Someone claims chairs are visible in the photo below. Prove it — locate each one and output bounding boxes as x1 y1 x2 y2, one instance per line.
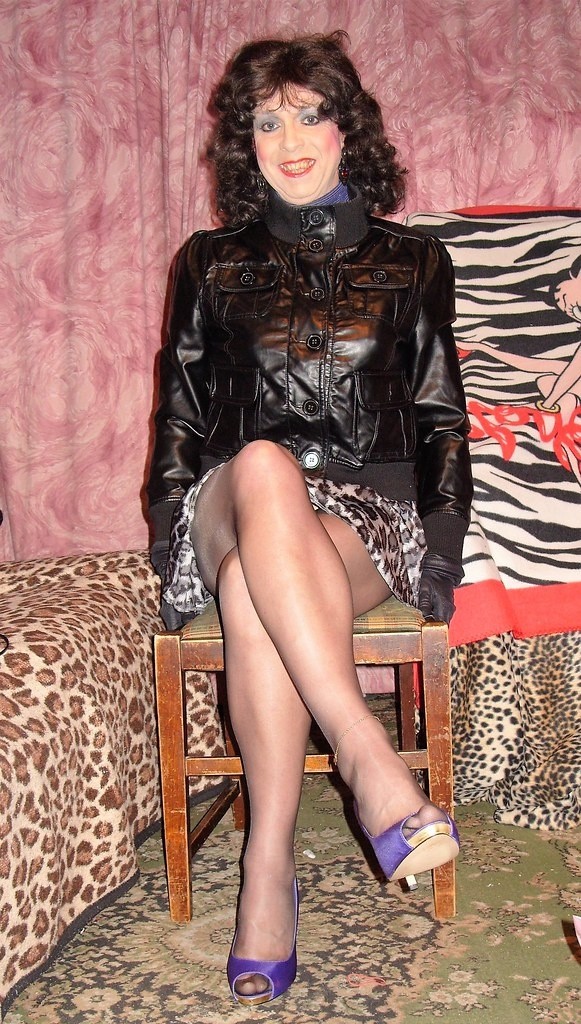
155 593 457 926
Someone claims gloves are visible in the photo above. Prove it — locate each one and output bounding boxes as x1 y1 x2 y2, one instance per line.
420 571 455 627
151 546 193 629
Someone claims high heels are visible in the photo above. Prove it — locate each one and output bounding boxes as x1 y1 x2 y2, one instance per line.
227 876 300 1005
354 797 459 890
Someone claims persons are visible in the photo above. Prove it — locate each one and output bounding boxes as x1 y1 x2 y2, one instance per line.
144 35 474 1008
